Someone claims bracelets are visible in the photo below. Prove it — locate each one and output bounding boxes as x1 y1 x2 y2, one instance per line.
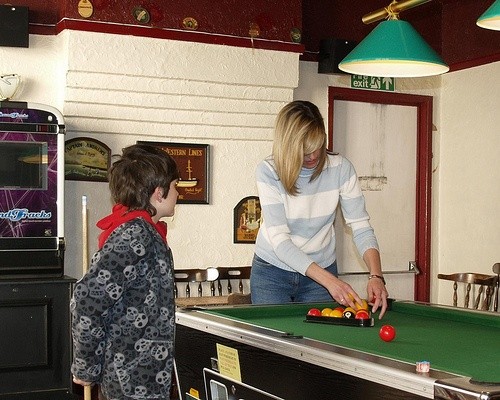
368 275 386 285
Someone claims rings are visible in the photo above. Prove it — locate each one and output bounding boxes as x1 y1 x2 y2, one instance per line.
340 299 345 302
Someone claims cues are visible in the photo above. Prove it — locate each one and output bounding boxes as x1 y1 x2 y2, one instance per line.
82 196 91 400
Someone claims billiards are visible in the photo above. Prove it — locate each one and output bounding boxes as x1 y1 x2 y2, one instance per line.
321 308 333 317
307 308 321 316
329 310 342 317
343 290 370 319
379 324 396 342
334 307 344 312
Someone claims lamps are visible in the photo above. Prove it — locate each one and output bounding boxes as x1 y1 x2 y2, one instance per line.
476 0 500 32
338 15 451 78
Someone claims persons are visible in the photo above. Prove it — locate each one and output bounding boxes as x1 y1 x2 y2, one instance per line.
249 101 389 319
70 144 178 400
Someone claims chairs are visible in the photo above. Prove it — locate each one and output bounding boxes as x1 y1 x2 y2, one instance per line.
438 263 500 312
174 267 251 298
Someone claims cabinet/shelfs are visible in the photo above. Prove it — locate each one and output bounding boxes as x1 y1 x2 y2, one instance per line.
0 274 78 400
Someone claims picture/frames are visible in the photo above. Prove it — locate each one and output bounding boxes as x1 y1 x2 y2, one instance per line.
136 141 210 204
65 137 111 182
233 196 262 244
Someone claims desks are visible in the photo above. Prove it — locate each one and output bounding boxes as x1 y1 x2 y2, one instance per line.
171 298 500 400
